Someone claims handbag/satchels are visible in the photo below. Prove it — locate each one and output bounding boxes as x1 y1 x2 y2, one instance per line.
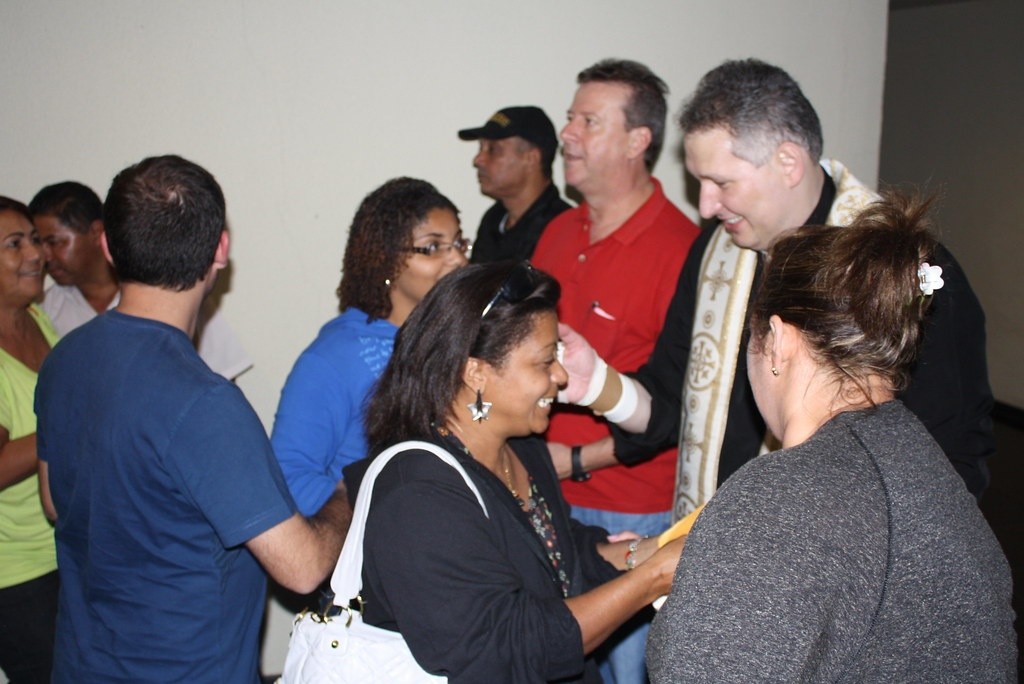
273 443 488 684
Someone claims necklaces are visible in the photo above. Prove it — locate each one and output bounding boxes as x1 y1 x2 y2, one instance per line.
502 458 523 507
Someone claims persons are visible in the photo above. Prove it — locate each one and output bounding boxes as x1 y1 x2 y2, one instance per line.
341 260 685 684
270 176 468 519
27 181 123 337
528 60 705 683
458 105 574 265
554 60 993 502
32 154 353 684
644 186 1018 684
1 193 62 684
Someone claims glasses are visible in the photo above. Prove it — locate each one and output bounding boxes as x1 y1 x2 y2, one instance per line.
400 238 470 258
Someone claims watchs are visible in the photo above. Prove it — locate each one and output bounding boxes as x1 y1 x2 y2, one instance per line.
570 446 592 482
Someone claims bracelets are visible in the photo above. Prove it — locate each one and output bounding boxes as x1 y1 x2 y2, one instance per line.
625 537 644 571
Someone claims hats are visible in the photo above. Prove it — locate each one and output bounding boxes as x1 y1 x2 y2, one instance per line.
459 106 558 154
